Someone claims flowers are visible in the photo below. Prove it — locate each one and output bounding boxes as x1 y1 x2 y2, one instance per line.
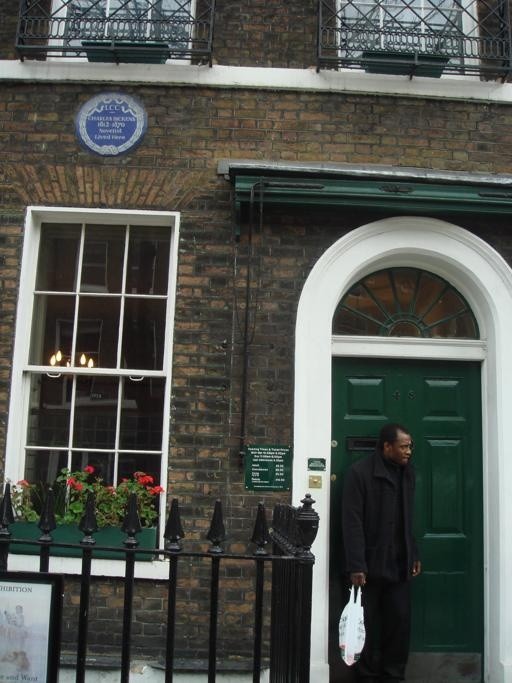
5 466 164 528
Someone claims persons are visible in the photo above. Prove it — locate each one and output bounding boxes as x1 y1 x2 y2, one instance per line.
329 423 421 683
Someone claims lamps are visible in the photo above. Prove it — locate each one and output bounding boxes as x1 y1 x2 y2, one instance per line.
47 350 94 377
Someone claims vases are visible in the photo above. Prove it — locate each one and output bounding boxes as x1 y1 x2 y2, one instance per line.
9 521 157 560
